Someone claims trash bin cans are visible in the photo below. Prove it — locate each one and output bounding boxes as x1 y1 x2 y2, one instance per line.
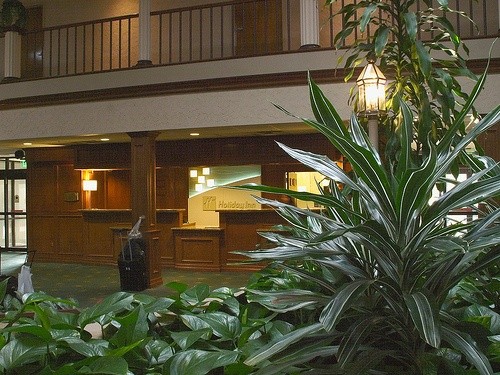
119 239 146 290
